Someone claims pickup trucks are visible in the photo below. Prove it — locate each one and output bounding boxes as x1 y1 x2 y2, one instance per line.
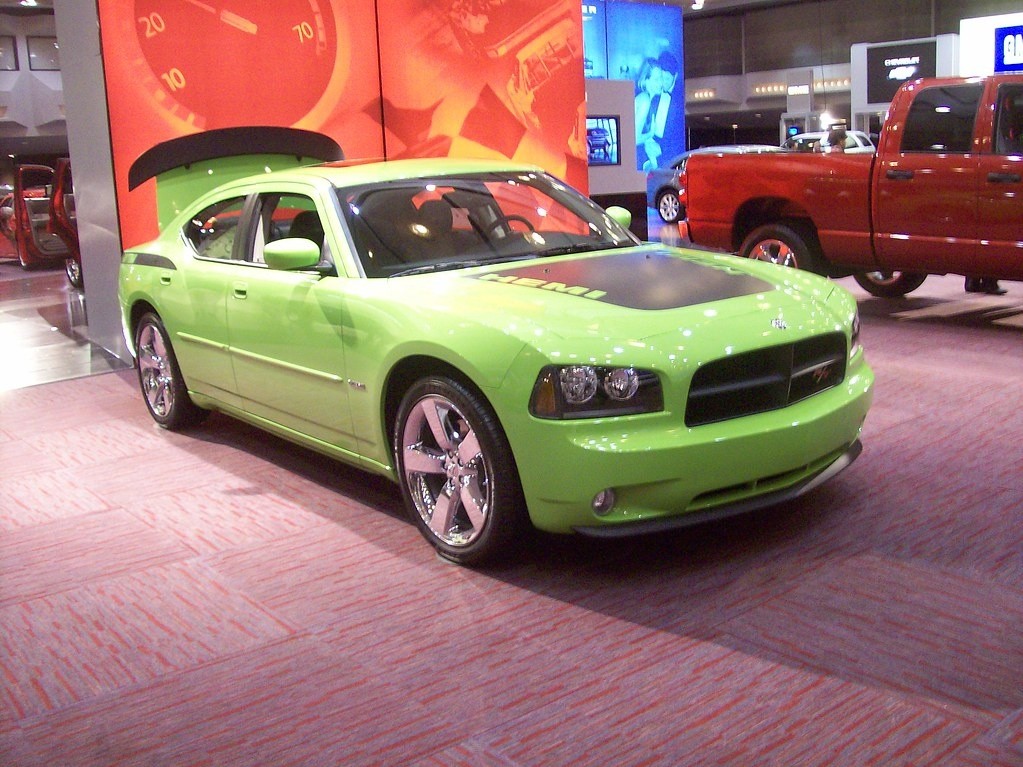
679 75 1023 281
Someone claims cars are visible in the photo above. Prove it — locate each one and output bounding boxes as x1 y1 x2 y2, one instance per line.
116 125 874 567
0 156 83 290
781 131 876 155
646 144 788 225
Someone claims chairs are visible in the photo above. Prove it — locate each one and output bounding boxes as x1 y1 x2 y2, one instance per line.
212 191 481 273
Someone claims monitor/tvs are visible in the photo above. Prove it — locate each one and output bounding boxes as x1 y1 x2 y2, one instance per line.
585 115 621 165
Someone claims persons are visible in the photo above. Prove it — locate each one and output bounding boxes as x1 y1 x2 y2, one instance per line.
964 276 1008 294
787 140 825 152
635 51 684 170
827 129 848 153
